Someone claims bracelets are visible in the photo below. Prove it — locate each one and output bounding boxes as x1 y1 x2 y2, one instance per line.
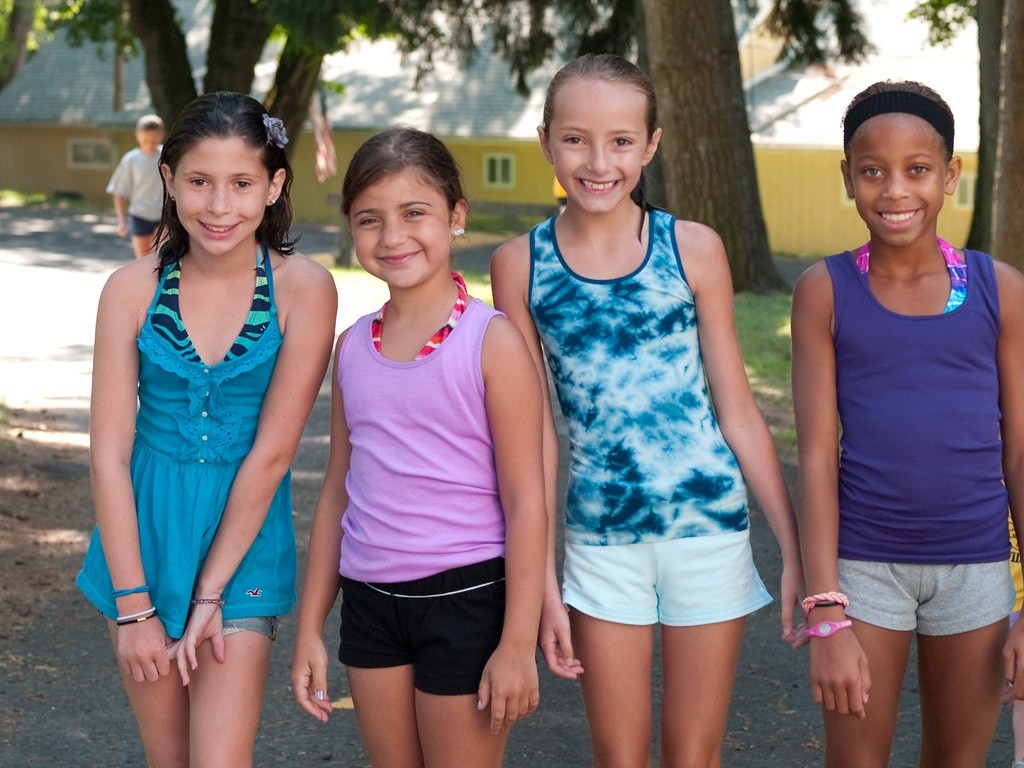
801 591 849 613
191 599 223 604
112 585 149 597
116 607 157 625
814 603 839 607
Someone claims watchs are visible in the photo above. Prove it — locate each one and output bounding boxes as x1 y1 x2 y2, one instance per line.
802 619 853 638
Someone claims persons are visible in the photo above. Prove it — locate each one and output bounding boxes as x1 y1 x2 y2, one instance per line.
792 81 1024 768
75 92 339 768
491 53 806 768
291 128 549 768
106 114 167 261
